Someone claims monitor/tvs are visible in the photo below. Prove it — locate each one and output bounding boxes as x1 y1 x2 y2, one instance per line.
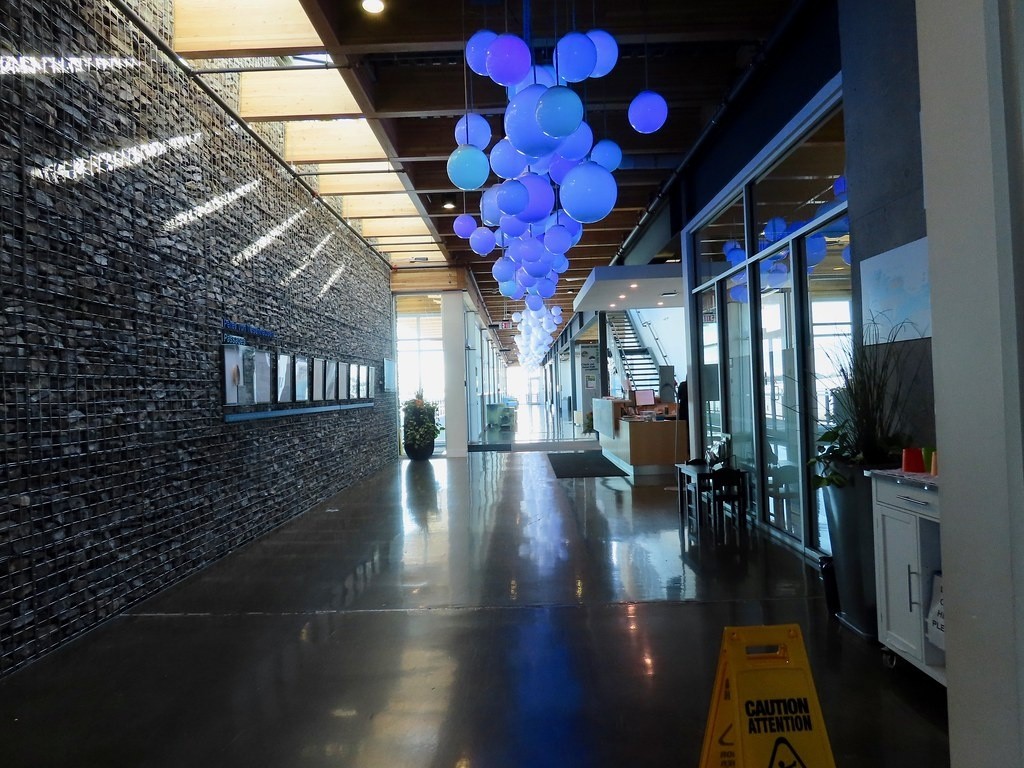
634 389 656 407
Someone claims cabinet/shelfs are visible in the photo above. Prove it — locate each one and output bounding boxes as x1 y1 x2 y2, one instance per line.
864 467 946 688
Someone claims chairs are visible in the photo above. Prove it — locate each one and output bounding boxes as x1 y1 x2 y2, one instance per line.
707 468 742 540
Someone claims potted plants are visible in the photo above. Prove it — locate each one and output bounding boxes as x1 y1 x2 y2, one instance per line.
401 388 446 461
784 307 933 630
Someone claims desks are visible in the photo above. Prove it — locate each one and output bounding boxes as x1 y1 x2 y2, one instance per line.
674 463 750 535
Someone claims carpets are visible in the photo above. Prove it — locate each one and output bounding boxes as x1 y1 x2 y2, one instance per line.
547 452 630 479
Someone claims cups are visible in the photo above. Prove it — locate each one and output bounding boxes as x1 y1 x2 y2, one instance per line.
902 448 925 473
931 452 937 476
921 447 936 472
665 407 669 415
